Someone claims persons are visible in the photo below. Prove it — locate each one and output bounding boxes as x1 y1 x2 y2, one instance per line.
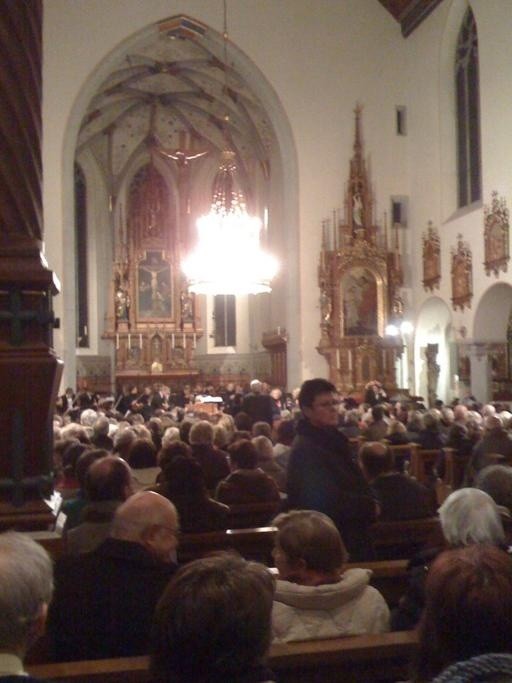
0 379 512 683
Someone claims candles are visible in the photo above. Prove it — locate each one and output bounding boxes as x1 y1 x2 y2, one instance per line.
116 334 119 350
128 333 130 349
193 333 196 348
183 335 186 348
172 334 174 348
140 334 142 349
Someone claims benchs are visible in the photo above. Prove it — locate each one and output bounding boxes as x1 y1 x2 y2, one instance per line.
349 435 504 506
0 501 447 682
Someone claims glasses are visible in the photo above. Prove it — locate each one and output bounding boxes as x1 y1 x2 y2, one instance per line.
314 400 339 407
159 524 184 539
